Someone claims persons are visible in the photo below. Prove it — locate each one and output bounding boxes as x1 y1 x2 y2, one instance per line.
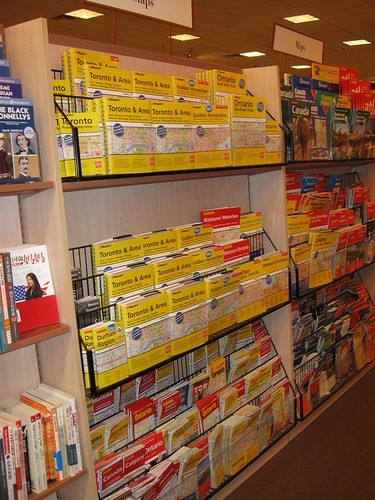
16 157 32 178
0 138 11 178
26 273 42 300
15 133 35 154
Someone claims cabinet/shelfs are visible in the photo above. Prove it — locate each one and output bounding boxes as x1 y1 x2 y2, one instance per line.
1 17 375 500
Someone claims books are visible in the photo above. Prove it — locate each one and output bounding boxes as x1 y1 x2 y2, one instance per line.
0 34 44 184
47 48 283 180
280 63 375 162
70 169 375 500
0 244 61 353
0 382 84 500
